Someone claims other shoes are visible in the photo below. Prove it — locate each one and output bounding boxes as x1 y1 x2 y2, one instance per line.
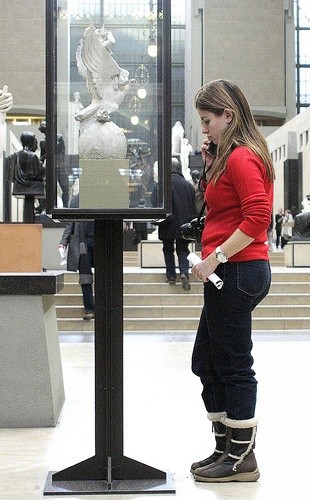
167 277 176 284
83 313 95 319
180 274 190 290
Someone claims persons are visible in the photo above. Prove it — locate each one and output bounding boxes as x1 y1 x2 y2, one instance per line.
58 192 95 320
11 131 45 186
39 121 70 207
150 157 196 290
190 80 275 481
275 208 293 248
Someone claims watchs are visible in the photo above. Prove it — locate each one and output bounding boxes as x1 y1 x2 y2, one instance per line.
215 246 228 263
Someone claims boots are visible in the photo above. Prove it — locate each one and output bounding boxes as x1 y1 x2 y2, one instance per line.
190 412 227 474
194 416 260 482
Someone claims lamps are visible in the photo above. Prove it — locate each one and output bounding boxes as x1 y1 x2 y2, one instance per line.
128 96 141 125
143 22 156 57
135 65 150 98
